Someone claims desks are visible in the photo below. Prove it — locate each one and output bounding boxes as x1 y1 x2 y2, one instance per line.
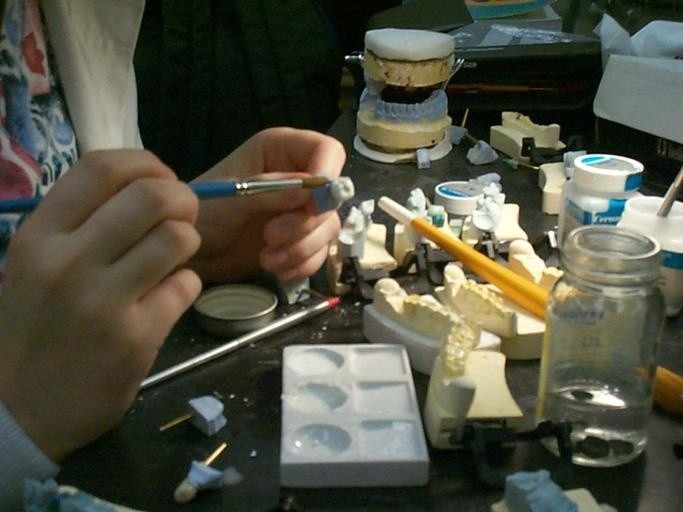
53 107 683 512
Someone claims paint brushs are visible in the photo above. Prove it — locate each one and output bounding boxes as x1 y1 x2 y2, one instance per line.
137 297 346 393
2 173 333 215
381 197 683 420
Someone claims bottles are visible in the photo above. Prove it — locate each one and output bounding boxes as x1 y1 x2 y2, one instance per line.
619 195 683 319
555 155 641 259
534 224 667 468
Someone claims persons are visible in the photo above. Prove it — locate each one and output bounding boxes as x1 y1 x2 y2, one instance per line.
0 2 346 512
